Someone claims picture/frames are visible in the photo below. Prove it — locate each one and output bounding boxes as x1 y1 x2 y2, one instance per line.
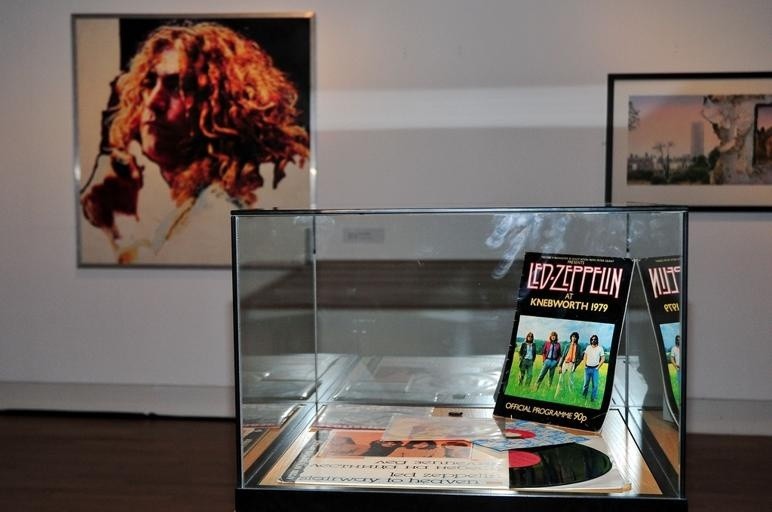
604 70 772 214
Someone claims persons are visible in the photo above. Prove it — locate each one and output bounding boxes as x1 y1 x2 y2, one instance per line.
359 438 403 457
531 331 561 391
440 440 470 458
553 332 581 401
401 439 438 459
518 331 536 388
328 434 360 456
580 335 605 402
670 331 682 388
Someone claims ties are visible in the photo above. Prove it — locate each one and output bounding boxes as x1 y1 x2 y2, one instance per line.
551 342 554 362
570 344 574 363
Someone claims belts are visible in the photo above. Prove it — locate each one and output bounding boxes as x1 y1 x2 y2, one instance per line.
586 365 598 368
